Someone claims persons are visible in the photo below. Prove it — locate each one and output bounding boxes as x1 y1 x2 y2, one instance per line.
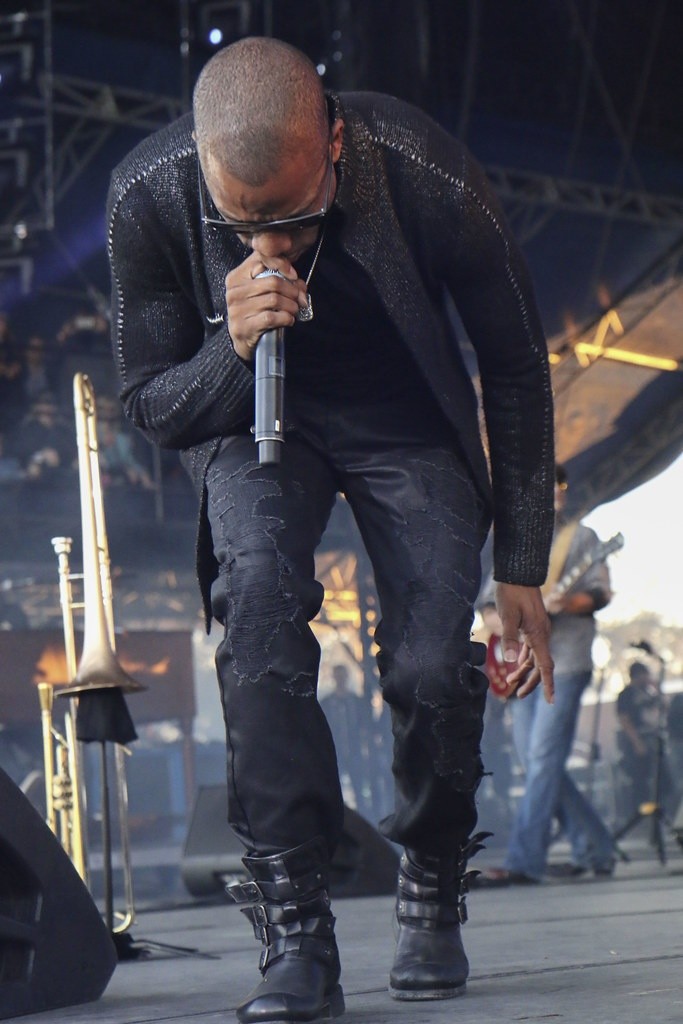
611 662 668 867
476 465 616 880
666 692 683 820
105 37 556 1023
320 665 365 811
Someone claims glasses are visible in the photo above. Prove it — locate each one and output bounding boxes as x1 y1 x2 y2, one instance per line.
195 126 331 233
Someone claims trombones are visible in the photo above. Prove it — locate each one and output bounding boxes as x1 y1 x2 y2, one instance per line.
37 369 136 940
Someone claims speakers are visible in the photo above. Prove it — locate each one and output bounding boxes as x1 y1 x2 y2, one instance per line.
182 784 400 906
0 768 118 1019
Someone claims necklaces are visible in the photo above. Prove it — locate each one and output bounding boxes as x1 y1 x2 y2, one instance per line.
297 230 326 323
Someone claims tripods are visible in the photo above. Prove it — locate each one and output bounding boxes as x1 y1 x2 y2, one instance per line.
613 642 682 867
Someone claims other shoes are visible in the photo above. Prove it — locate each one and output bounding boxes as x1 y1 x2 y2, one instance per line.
478 868 540 884
545 860 614 877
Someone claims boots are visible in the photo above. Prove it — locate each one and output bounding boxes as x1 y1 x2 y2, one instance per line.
225 833 345 1024
389 830 496 999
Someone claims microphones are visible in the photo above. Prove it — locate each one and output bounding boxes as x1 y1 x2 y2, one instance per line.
251 269 293 469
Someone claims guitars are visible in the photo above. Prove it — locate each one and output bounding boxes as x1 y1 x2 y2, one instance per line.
483 531 625 697
